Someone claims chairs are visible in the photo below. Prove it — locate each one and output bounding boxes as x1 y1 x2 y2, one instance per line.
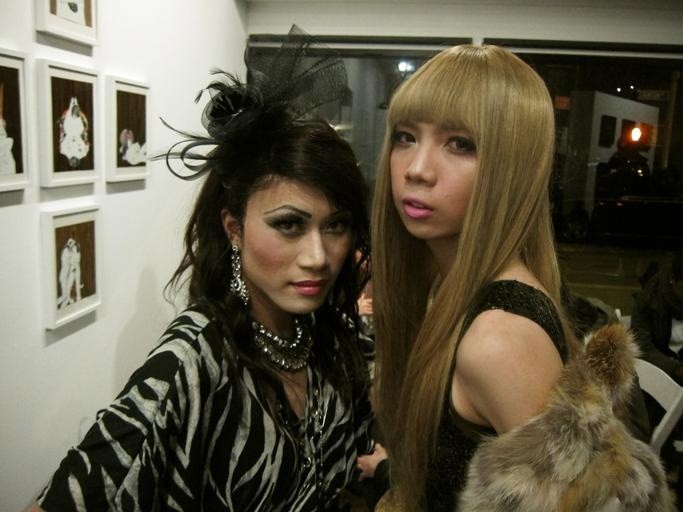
632 357 683 457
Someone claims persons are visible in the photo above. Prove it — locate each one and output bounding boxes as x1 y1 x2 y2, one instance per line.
19 116 383 512
57 238 77 305
355 437 390 495
60 98 90 167
366 41 677 511
354 243 375 316
59 242 83 308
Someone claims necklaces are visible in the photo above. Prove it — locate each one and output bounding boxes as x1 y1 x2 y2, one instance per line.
247 313 324 467
